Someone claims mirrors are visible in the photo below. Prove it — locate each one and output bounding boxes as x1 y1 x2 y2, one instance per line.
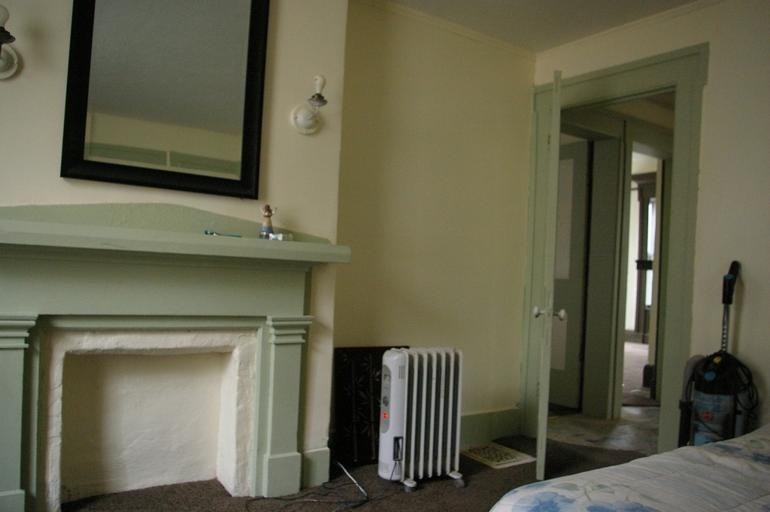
59 0 270 201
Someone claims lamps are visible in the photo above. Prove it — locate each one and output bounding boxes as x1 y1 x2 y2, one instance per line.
0 4 19 80
290 74 329 135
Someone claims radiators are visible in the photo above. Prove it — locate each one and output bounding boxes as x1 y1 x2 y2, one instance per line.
378 347 463 491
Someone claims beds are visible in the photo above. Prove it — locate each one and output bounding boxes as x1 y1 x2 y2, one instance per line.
491 424 770 512
